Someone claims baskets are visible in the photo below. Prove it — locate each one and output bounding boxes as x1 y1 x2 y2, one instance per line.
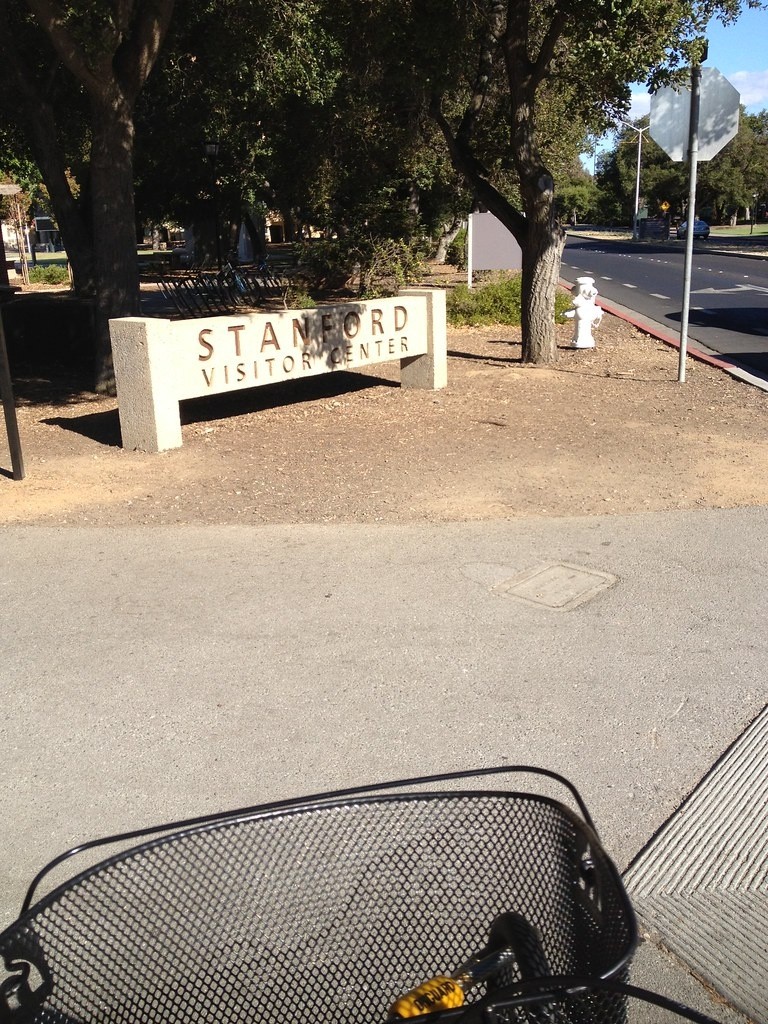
1 766 641 1021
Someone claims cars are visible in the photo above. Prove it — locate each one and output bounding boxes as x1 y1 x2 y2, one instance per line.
677 220 710 239
